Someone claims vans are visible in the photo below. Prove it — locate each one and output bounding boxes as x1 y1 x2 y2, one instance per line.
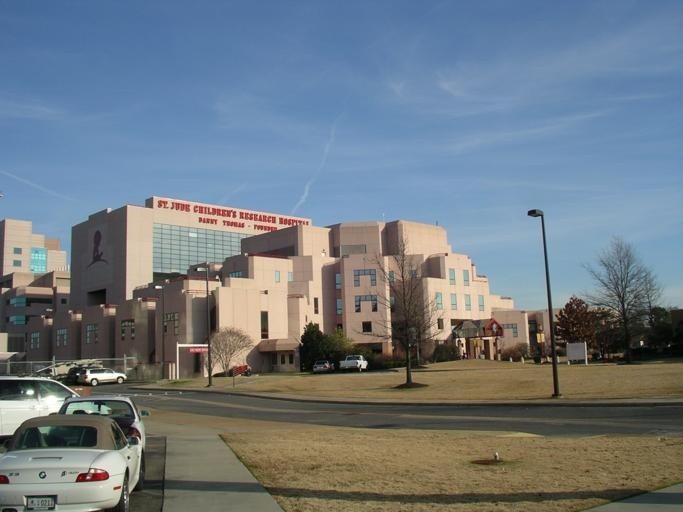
0 375 81 436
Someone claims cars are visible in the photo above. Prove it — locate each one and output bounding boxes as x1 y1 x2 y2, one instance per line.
312 360 334 374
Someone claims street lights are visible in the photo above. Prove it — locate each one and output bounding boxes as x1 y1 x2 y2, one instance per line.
161 278 169 379
193 267 213 387
537 323 543 357
527 208 562 397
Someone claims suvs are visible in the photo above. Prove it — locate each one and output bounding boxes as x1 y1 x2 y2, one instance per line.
66 366 100 385
47 394 149 453
76 367 127 387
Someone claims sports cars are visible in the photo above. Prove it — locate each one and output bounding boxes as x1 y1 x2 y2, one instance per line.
0 415 145 511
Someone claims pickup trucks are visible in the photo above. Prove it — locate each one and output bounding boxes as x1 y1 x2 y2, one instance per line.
338 354 368 373
